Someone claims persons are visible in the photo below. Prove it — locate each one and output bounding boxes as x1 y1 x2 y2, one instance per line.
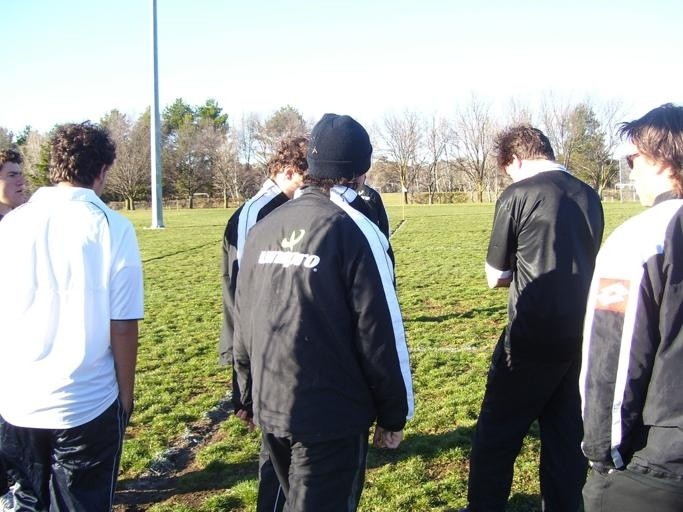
355 173 389 235
0 125 142 512
220 134 309 512
233 113 415 511
1 149 24 511
578 103 683 512
458 126 604 512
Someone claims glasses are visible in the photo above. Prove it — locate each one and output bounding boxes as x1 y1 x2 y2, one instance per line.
626 152 640 169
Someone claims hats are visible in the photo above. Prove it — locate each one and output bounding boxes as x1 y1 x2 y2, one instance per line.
307 113 372 179
